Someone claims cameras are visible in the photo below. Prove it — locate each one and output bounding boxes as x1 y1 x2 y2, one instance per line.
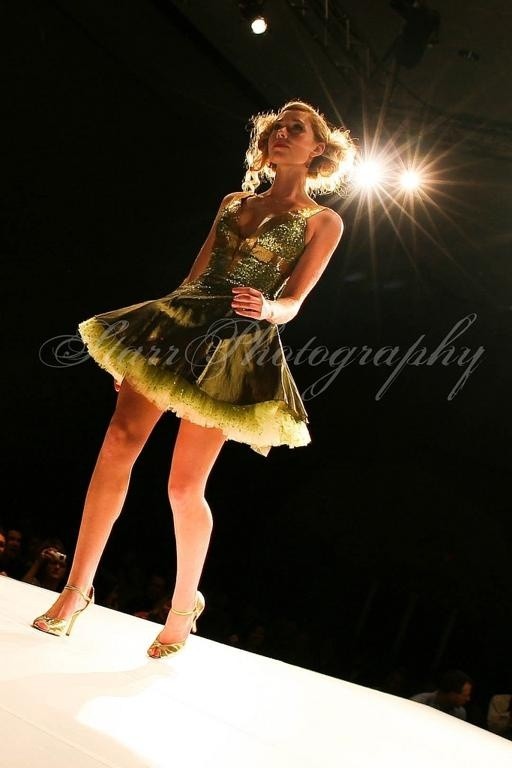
54 551 67 563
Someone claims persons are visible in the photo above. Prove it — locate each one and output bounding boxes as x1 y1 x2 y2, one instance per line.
32 96 355 660
2 526 512 739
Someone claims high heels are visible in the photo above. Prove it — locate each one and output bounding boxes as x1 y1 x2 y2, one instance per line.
34 584 95 636
148 591 205 659
